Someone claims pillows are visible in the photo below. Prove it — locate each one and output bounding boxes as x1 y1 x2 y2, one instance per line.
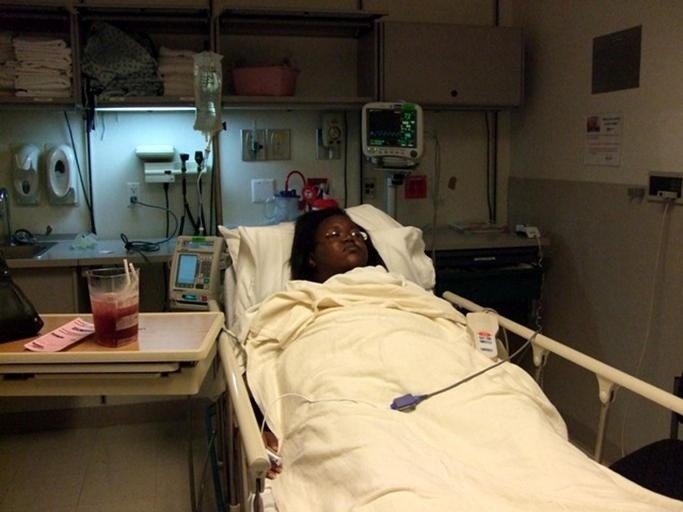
238 203 436 302
216 224 257 308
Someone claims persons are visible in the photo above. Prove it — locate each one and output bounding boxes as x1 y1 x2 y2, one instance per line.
242 208 465 480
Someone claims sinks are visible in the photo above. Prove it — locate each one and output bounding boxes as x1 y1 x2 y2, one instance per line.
0 242 58 259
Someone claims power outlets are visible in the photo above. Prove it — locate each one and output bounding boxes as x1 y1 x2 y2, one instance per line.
644 170 683 202
251 177 275 203
127 183 142 208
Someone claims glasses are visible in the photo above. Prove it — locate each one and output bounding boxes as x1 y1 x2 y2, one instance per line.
323 230 368 241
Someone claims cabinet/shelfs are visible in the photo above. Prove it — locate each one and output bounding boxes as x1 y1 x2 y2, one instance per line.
383 18 528 110
421 227 554 326
2 1 391 110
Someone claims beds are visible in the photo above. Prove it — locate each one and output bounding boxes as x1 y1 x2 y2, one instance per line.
205 202 683 512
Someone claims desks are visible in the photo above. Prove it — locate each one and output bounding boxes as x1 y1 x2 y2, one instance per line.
0 310 225 409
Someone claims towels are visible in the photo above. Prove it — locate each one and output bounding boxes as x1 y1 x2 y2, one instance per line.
158 44 197 98
0 36 73 97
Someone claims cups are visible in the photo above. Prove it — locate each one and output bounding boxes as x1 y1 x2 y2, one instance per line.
262 189 300 224
85 266 142 350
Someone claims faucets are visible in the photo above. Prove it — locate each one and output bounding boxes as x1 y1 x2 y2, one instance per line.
0 187 13 243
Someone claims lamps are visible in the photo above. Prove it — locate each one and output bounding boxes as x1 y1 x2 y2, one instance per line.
96 105 197 115
